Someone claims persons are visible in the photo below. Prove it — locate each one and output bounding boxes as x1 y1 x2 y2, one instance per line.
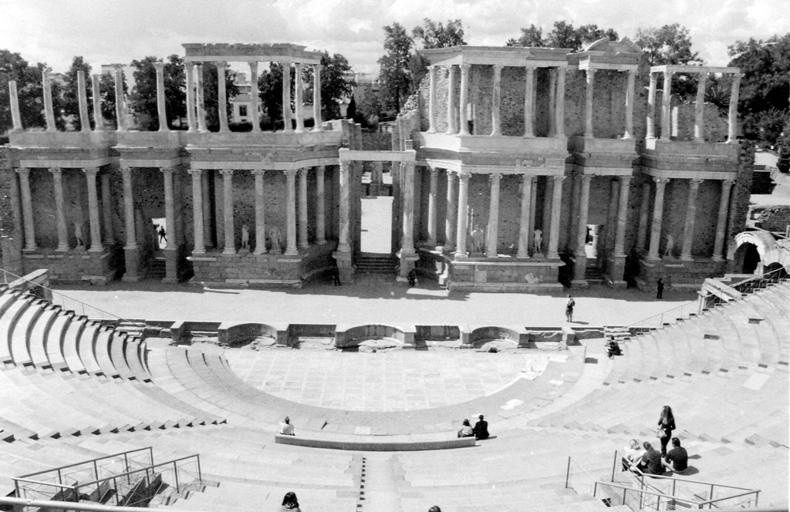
159 226 166 243
657 278 664 299
458 420 474 437
607 336 621 356
280 416 294 435
407 269 416 287
280 492 301 512
566 294 574 322
622 406 687 478
474 415 489 439
332 264 341 286
